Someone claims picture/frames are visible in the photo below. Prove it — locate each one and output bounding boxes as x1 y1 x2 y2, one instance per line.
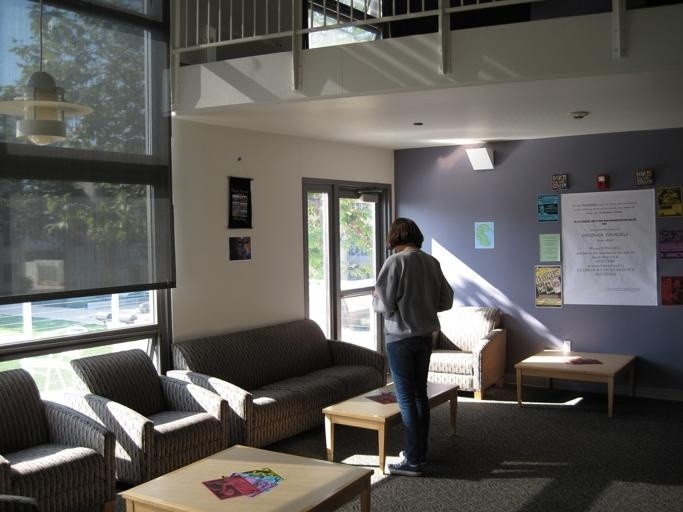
533 264 563 309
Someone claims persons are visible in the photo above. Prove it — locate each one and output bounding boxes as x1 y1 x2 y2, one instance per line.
372 217 454 476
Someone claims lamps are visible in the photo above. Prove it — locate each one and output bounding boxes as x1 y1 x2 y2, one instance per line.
0 0 94 147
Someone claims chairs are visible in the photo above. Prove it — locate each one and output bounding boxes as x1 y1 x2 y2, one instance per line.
0 368 117 511
59 347 232 486
426 305 508 400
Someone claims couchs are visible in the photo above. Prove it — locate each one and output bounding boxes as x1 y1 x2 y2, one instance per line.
169 318 388 449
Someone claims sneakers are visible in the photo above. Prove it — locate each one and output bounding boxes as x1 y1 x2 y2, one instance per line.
388 451 427 476
